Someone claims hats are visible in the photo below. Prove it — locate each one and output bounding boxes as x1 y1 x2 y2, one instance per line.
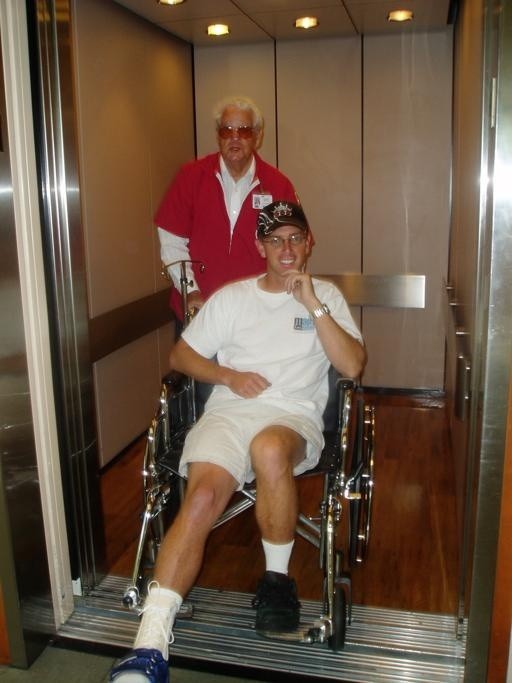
258 201 308 238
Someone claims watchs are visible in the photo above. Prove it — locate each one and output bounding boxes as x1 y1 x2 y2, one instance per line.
305 302 331 320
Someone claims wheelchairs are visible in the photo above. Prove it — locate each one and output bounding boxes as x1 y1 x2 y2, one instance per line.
121 303 378 652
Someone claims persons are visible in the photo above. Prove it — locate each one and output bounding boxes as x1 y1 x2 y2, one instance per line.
101 199 367 683
154 96 314 530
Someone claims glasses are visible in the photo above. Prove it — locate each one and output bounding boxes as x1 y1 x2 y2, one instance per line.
217 125 254 140
262 233 307 246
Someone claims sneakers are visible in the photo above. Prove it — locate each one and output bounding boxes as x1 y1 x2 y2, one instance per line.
254 572 300 635
